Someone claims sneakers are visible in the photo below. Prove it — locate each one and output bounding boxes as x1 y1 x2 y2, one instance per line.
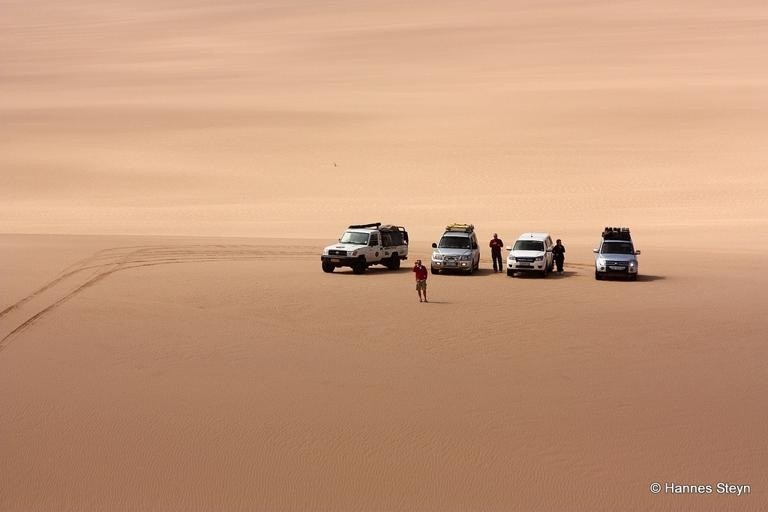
556 270 564 275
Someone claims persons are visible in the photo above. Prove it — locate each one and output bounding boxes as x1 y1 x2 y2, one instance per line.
550 238 565 275
411 259 428 303
488 232 504 273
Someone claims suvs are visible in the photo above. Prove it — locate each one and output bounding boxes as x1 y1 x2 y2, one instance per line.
505 232 554 278
431 230 480 274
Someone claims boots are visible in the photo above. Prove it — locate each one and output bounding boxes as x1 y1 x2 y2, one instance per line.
419 299 427 302
493 270 501 273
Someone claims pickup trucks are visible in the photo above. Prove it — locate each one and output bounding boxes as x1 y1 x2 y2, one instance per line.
593 238 641 280
321 228 408 274
